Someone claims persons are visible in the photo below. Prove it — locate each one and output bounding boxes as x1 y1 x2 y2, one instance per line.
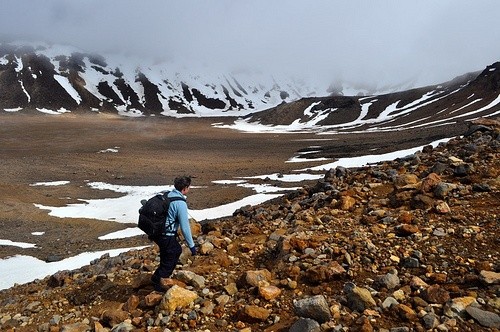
150 175 199 295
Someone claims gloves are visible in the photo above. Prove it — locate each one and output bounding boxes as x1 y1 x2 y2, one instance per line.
191 247 196 256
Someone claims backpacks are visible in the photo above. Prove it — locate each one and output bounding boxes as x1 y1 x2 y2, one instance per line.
138 191 187 239
